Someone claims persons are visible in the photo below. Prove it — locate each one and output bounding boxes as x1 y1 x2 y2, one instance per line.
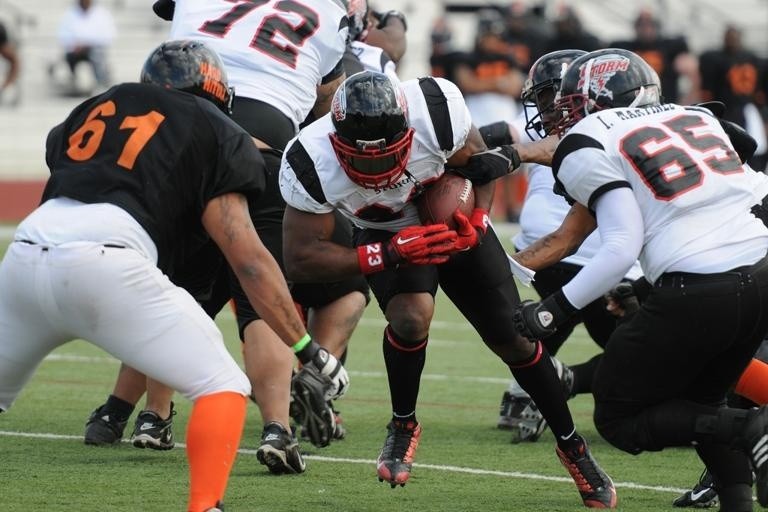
0 17 20 94
277 70 623 510
2 34 354 512
44 0 117 102
512 48 767 510
81 3 768 474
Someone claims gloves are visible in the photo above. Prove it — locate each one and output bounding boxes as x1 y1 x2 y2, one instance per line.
356 209 489 277
455 144 520 187
510 286 576 344
296 339 350 401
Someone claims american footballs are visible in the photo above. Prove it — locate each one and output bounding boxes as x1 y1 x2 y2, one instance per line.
417 174 476 230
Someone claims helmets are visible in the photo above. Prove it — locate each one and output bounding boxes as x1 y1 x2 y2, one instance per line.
328 70 416 191
518 50 661 140
139 39 236 116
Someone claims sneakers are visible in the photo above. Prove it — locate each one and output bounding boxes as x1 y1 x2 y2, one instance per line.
255 364 346 473
725 404 768 510
672 466 720 509
83 402 126 449
375 417 421 488
131 403 175 451
554 432 617 509
499 357 574 444
